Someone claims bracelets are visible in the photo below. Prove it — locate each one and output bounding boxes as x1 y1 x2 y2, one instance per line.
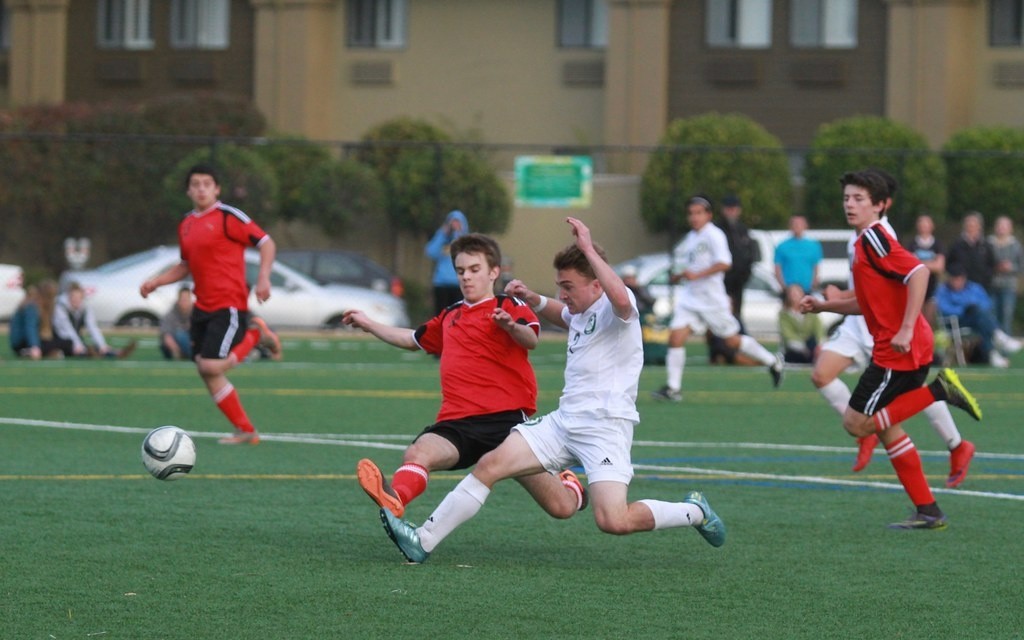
532 295 547 312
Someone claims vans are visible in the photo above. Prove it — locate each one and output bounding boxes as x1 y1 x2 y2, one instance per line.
745 227 855 298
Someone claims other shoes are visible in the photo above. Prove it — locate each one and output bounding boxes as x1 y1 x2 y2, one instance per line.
993 328 1022 351
989 351 1009 368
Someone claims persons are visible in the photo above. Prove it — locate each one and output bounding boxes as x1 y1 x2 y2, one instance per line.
619 264 655 315
9 282 136 360
799 169 981 529
140 166 281 445
343 234 589 519
159 286 195 360
812 169 974 487
425 211 468 315
649 197 783 401
779 283 826 365
907 214 945 320
706 198 761 367
774 215 822 308
379 216 725 563
935 263 1022 368
988 214 1024 333
947 211 997 294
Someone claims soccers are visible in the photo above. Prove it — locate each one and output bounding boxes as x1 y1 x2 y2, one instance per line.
140 424 197 482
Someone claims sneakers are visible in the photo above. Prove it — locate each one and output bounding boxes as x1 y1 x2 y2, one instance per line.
770 352 785 389
936 368 983 422
886 506 948 532
379 507 430 565
851 432 880 472
559 469 589 511
651 385 683 402
357 458 404 518
684 489 726 547
218 430 260 445
945 440 975 486
247 317 281 359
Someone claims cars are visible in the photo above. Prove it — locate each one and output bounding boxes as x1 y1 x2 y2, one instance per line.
612 251 845 346
60 242 410 335
0 265 26 321
277 247 404 299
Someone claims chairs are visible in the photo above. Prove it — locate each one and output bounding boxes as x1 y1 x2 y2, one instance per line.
938 311 974 367
